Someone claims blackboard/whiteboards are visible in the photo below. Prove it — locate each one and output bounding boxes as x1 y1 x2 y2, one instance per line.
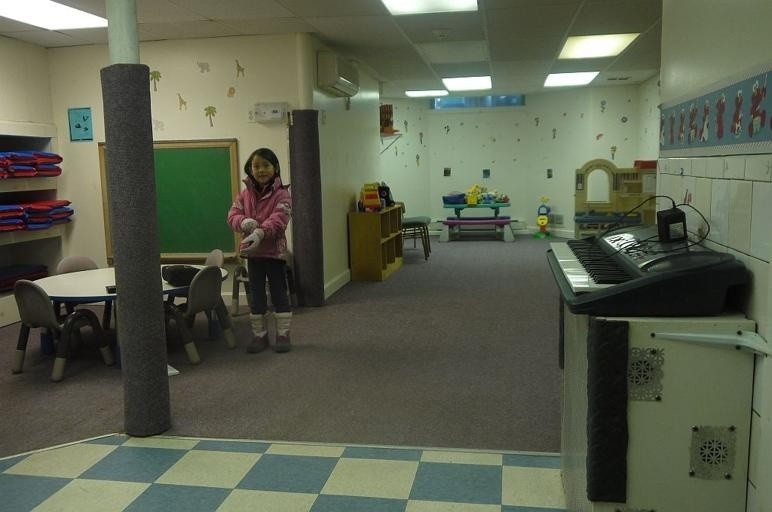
98 138 242 266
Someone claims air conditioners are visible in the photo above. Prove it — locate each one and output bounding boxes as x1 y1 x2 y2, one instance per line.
317 51 360 97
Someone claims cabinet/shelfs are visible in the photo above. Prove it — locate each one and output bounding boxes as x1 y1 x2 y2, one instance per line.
574 159 656 225
349 204 403 281
0 119 67 326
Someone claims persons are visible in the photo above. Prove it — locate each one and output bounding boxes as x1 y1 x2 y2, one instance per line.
226 148 293 354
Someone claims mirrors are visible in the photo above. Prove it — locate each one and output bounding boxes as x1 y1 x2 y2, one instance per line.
584 165 614 205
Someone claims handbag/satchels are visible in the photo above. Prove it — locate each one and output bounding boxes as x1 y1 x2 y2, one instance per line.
162 264 202 287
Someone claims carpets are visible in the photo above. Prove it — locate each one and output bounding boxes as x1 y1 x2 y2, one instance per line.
0 433 569 512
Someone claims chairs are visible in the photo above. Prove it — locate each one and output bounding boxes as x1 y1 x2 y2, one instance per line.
395 202 431 261
14 249 296 382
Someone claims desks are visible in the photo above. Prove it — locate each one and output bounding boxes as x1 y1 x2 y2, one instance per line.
444 203 511 239
574 215 641 238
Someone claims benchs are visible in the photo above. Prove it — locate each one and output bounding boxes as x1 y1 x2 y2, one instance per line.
439 216 518 242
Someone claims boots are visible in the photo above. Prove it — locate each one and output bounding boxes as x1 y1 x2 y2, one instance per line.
273 311 292 352
247 313 268 353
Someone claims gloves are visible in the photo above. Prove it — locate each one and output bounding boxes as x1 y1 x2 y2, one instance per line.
241 229 265 255
241 218 259 232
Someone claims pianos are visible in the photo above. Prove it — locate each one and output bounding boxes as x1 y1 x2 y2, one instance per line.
545 223 752 317
